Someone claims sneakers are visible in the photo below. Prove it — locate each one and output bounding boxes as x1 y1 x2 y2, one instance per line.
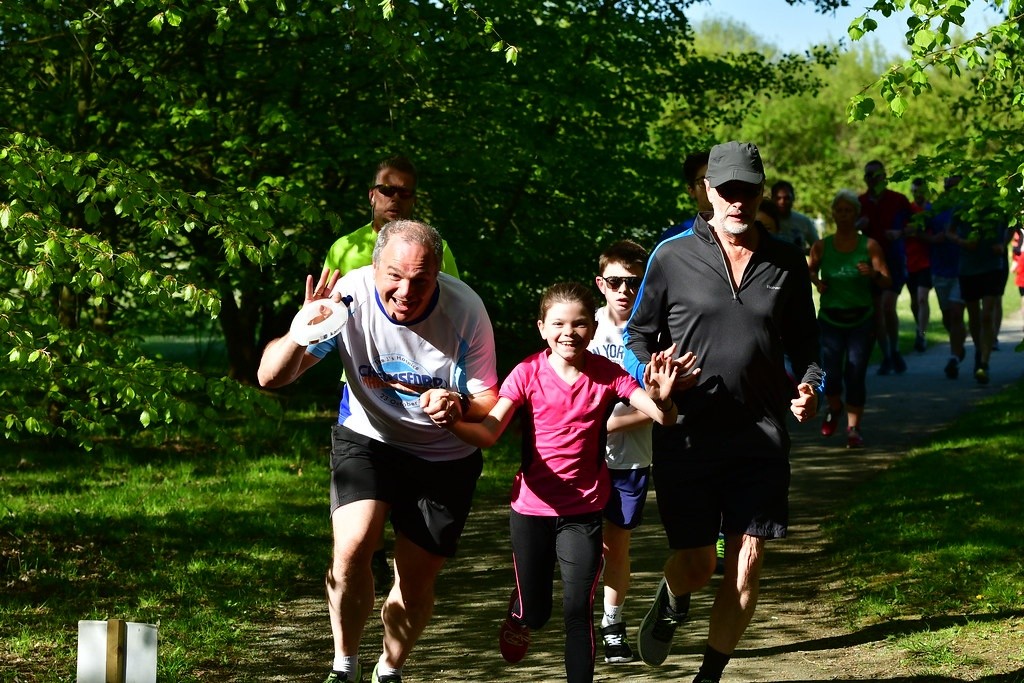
598 621 635 663
371 662 403 683
498 586 531 664
636 576 681 668
821 414 838 438
845 426 866 449
322 663 363 683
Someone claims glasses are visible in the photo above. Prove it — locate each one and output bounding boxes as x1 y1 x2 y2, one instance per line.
695 176 705 189
371 184 415 200
602 276 642 290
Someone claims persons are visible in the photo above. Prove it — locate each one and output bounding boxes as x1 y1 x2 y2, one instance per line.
257 219 497 683
429 281 678 683
622 139 829 683
586 240 656 663
319 157 460 596
652 151 1024 575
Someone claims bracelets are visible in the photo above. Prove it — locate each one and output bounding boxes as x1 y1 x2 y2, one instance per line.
658 398 673 412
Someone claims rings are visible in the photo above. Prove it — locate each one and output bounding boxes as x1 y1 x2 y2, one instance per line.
447 414 453 423
316 292 322 296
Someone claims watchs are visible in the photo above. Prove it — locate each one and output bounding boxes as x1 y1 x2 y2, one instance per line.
454 392 471 415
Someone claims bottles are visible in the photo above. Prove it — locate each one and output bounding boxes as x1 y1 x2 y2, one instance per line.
290 294 353 348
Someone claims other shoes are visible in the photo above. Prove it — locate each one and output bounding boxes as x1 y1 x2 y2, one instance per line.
972 352 981 374
1014 338 1024 353
716 538 725 572
943 358 959 381
991 339 1000 351
974 363 990 386
917 338 927 352
891 351 908 375
876 359 893 376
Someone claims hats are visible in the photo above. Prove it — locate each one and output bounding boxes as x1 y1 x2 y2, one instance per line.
705 140 767 189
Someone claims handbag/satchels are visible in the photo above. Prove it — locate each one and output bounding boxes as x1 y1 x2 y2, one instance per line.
820 306 871 324
